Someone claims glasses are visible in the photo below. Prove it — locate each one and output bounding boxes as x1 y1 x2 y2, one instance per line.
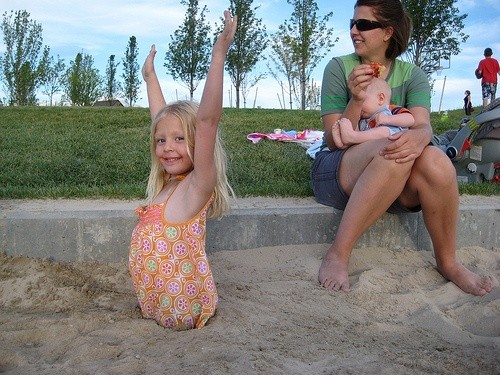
349 18 386 32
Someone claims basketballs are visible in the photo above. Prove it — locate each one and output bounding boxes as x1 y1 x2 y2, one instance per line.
475 69 484 78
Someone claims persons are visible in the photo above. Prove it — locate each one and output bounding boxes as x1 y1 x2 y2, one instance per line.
464 90 472 115
128 10 238 332
475 48 500 108
332 77 415 149
310 0 494 297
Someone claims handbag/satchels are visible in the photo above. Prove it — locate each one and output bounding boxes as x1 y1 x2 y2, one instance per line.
470 108 474 112
